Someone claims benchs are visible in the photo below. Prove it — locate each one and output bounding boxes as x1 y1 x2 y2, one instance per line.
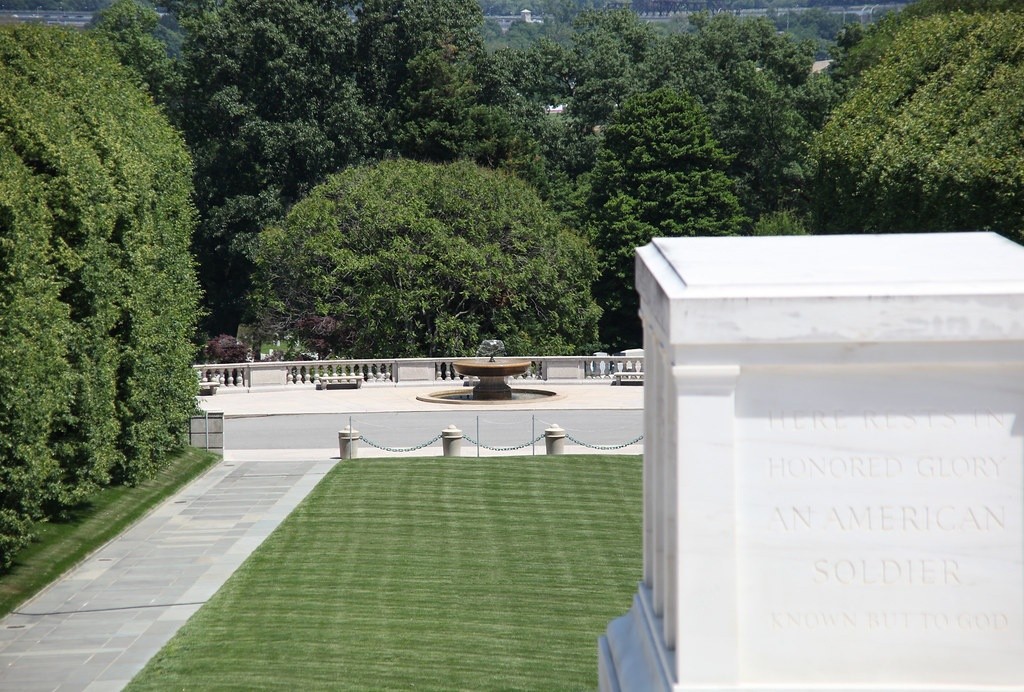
316 375 364 390
199 382 221 396
614 372 644 385
